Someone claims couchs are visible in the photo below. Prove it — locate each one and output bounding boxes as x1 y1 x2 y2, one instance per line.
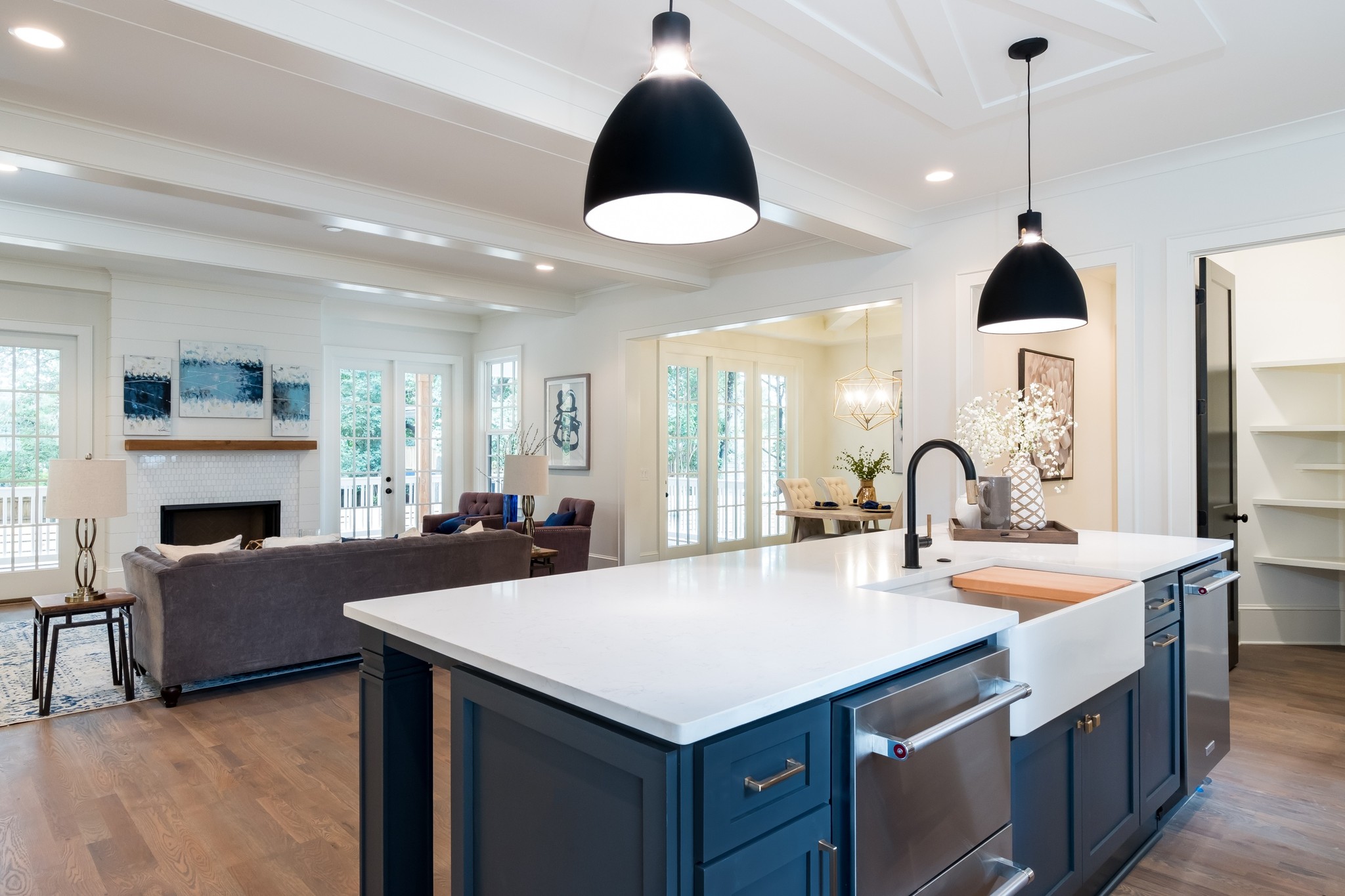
503 496 596 579
420 491 510 540
122 524 536 708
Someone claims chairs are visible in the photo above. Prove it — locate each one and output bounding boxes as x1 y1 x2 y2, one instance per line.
776 476 848 541
814 476 888 536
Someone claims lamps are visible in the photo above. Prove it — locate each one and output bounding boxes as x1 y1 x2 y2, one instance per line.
974 36 1091 335
41 452 129 603
831 307 902 431
500 452 550 553
580 0 761 244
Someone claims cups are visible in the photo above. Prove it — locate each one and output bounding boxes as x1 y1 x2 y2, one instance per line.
977 475 1012 530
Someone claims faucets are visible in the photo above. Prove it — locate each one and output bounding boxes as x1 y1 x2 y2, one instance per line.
901 438 980 570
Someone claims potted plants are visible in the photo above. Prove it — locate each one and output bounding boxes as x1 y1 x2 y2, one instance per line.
831 445 894 505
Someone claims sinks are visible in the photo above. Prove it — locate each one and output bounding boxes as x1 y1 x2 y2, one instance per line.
855 556 1145 738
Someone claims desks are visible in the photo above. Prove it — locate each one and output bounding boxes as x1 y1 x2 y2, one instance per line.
29 587 140 717
336 522 1242 896
774 500 899 535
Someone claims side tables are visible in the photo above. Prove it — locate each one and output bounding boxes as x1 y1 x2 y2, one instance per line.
528 545 559 579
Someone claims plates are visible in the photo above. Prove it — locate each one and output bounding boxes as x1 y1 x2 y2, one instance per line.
812 505 837 509
850 502 858 505
862 508 895 512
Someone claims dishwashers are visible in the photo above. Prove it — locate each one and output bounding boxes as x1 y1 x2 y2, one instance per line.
839 643 1036 896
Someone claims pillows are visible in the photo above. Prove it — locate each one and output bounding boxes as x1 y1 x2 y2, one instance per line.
435 512 489 532
261 533 344 550
543 511 578 527
151 532 246 563
461 520 485 535
395 525 422 539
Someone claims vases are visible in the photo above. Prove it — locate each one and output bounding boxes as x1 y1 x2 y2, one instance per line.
997 450 1049 530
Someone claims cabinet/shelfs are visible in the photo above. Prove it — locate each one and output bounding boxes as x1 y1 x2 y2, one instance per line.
1245 356 1345 576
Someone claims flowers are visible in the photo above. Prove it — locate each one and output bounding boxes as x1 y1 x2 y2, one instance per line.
953 381 1079 494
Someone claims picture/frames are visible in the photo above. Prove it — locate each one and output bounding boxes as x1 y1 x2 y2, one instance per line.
541 372 593 471
1013 346 1075 484
890 368 905 475
